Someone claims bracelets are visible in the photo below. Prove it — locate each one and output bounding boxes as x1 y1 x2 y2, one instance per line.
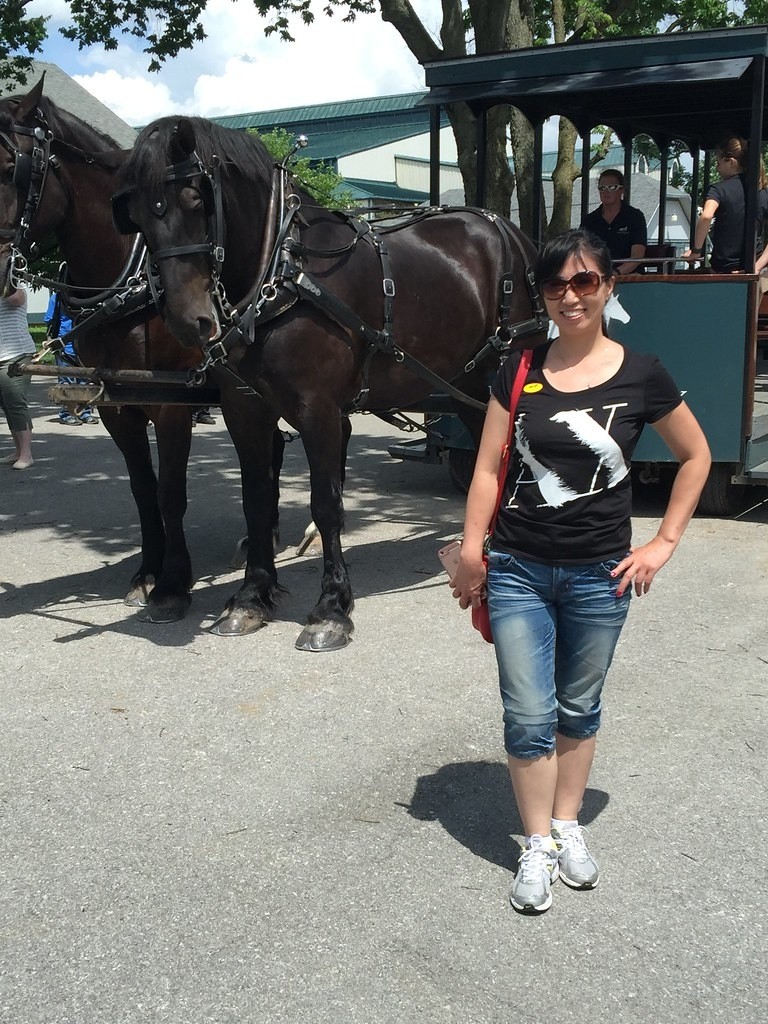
692 245 703 254
613 268 621 275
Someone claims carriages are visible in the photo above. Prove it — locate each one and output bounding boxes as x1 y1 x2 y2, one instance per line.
0 19 768 655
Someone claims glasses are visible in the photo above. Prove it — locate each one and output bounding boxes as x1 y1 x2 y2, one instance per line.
598 184 624 191
539 271 606 300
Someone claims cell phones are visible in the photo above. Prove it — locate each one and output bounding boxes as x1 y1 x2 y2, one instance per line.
437 541 487 606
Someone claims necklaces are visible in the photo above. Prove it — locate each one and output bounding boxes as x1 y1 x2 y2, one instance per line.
570 368 593 388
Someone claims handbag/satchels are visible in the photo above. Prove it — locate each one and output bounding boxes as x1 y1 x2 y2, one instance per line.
472 535 494 643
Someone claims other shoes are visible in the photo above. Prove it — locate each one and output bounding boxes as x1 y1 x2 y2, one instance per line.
193 414 216 427
59 414 99 426
13 459 34 469
0 453 18 462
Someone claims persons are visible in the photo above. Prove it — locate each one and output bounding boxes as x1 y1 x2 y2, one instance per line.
680 137 768 275
43 293 99 425
449 229 712 914
0 283 37 469
579 168 647 275
192 407 216 427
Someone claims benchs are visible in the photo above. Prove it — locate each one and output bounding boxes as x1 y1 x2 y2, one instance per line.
644 245 677 274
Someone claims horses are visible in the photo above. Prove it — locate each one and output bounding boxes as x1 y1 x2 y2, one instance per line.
0 71 541 654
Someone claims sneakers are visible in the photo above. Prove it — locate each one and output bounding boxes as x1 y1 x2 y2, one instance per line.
510 834 561 914
550 825 600 891
